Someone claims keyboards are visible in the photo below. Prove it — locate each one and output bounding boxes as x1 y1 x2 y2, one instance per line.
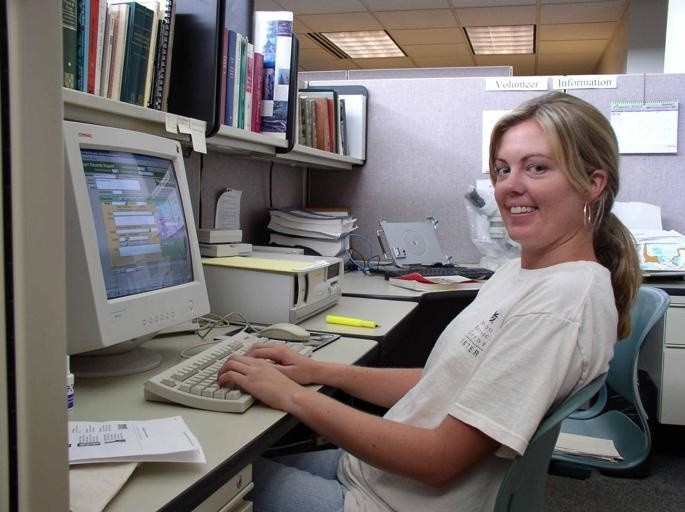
387 267 495 280
144 329 312 413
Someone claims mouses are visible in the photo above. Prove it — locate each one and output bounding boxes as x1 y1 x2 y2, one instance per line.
256 323 311 341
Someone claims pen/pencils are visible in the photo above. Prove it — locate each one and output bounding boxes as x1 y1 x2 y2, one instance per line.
326 315 382 329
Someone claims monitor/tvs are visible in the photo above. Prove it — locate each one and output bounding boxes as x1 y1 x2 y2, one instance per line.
62 119 212 378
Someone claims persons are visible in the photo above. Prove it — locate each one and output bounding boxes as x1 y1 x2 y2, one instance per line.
211 88 645 512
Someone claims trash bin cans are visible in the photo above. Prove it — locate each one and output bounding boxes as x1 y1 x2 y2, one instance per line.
589 370 658 479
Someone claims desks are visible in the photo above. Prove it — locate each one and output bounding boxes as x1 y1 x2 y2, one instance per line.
335 264 489 297
302 292 430 361
70 340 387 510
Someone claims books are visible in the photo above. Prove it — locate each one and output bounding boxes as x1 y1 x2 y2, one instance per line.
220 27 263 133
298 88 345 155
262 196 364 276
61 0 176 112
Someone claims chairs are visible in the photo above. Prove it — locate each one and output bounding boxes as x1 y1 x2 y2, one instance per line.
498 360 607 510
558 281 672 477
